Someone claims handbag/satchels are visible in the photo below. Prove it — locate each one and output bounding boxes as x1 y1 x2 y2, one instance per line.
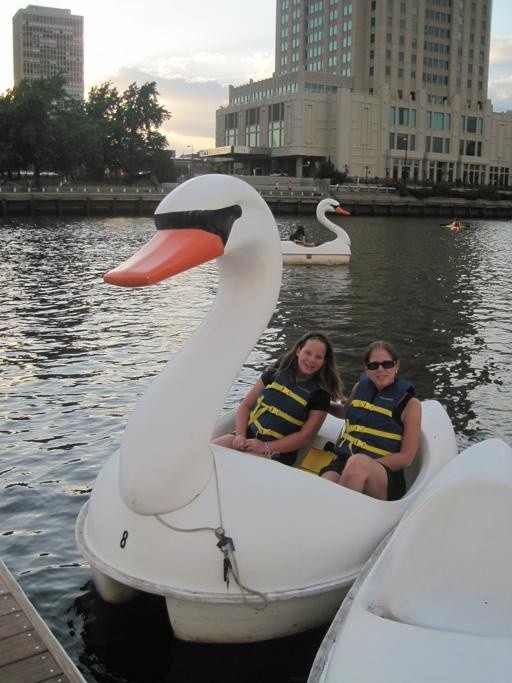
300 448 337 475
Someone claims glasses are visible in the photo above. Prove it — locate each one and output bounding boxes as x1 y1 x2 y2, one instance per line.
365 361 396 370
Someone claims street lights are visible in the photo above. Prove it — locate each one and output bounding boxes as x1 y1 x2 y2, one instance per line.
344 158 349 177
402 136 408 169
187 144 194 157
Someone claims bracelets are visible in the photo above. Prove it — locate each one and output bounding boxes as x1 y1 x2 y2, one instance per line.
264 441 270 457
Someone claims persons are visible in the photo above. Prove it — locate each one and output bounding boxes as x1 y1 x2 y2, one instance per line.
318 340 422 501
288 181 292 190
211 331 347 465
294 225 314 246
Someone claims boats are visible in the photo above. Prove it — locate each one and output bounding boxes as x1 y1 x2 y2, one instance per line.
305 438 511 682
277 196 355 266
65 174 462 653
